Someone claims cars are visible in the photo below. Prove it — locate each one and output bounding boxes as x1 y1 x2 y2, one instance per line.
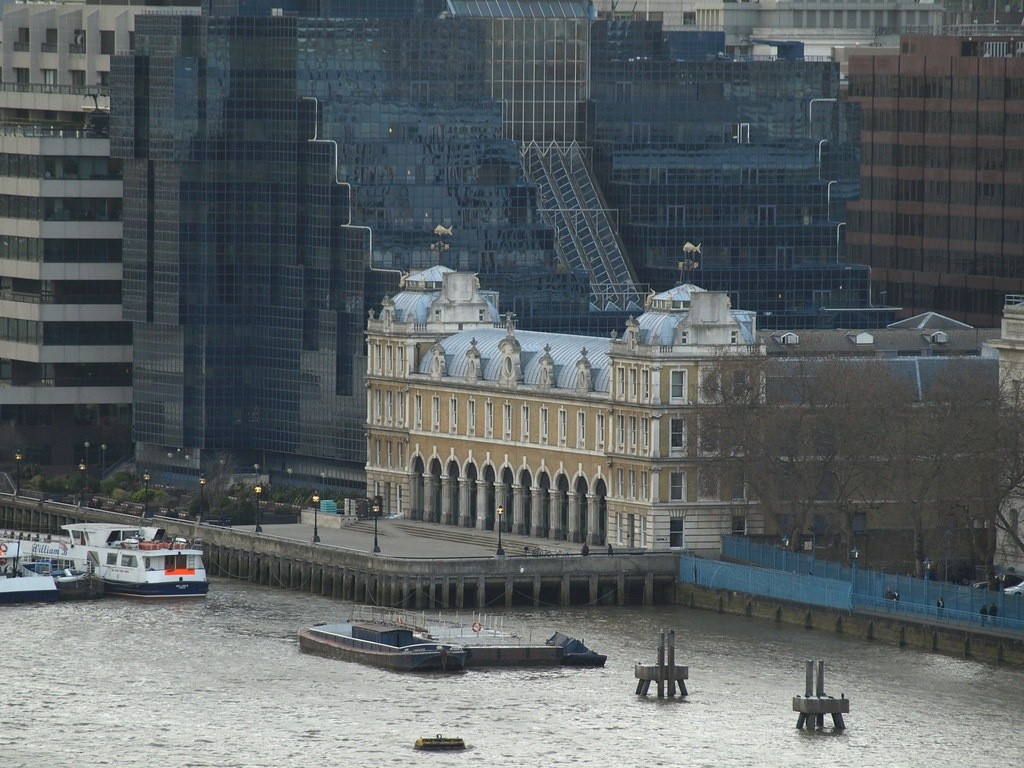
1004 581 1024 598
972 574 1024 591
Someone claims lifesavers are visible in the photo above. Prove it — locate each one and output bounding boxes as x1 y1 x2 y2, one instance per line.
0 543 8 552
472 623 481 632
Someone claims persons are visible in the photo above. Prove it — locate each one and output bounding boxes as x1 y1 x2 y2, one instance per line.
165 508 179 518
937 595 945 620
86 497 104 508
884 586 901 613
581 542 592 555
607 543 614 555
522 544 529 556
980 602 999 628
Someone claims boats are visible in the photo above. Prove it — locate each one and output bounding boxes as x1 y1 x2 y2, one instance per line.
0 539 105 604
0 521 212 598
298 621 467 671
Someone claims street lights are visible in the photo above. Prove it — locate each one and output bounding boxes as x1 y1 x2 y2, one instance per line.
15 451 22 496
143 473 151 518
255 486 262 532
101 444 107 480
372 501 381 553
84 442 90 479
495 506 505 555
78 463 86 507
199 474 206 521
254 464 260 485
185 455 191 487
287 468 293 503
312 494 320 542
